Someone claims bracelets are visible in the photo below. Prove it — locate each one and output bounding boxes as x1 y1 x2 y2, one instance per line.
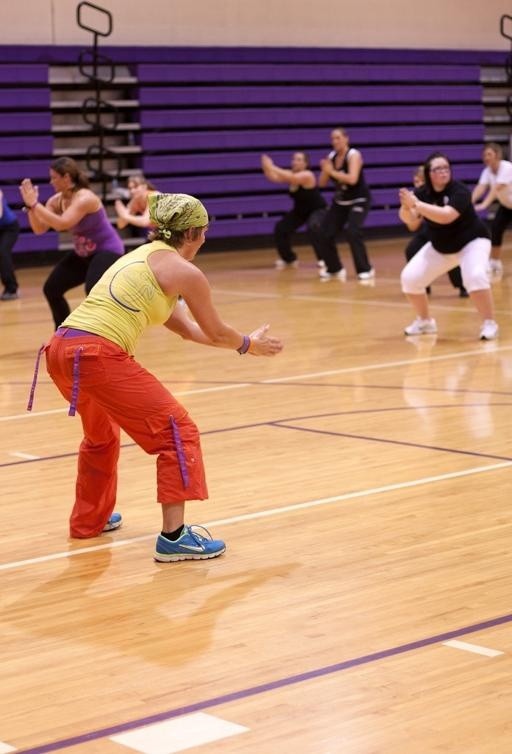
237 335 250 356
31 200 40 209
411 199 420 209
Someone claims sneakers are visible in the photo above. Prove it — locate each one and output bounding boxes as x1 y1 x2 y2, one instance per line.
404 317 437 335
275 258 375 280
100 513 122 530
0 292 18 300
480 321 498 340
153 527 226 562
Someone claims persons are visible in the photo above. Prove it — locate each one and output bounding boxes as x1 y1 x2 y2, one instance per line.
0 190 20 301
114 173 184 302
399 152 500 339
319 128 375 280
27 189 283 561
19 158 124 331
261 150 328 267
470 143 512 272
405 166 469 297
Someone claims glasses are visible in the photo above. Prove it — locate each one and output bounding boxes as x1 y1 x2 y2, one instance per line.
434 167 451 173
